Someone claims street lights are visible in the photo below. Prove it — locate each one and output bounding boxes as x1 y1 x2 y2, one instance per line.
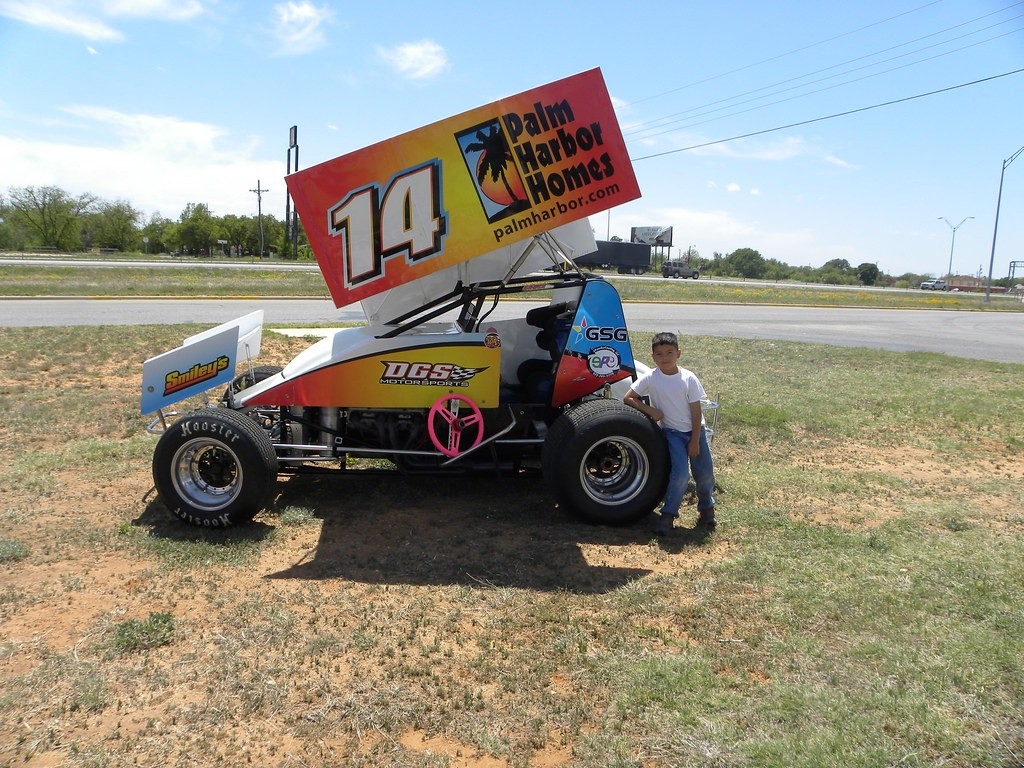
937 216 976 293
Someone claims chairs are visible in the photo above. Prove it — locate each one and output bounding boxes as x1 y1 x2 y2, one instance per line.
503 300 578 404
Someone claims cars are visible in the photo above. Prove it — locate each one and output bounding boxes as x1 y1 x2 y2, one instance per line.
132 265 685 533
920 279 946 291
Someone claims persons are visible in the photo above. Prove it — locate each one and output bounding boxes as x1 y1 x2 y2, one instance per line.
623 332 717 534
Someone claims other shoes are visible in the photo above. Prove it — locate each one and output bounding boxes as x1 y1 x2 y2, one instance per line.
656 515 675 535
701 508 717 525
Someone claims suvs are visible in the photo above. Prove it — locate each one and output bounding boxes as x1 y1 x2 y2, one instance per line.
661 260 700 280
